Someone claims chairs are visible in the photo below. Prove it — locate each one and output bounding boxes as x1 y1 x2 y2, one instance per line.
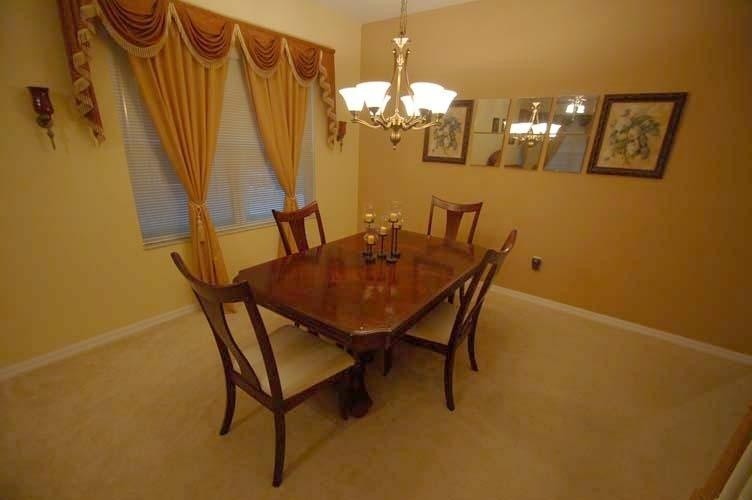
428 194 482 305
171 252 358 487
271 200 343 356
384 229 518 411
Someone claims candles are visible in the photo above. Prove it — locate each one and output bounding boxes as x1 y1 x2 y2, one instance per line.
365 213 400 244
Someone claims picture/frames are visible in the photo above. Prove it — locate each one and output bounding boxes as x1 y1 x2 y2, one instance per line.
586 92 690 179
420 99 474 163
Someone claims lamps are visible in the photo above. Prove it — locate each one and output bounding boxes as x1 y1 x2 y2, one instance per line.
338 0 458 152
337 120 347 152
26 85 56 152
509 100 562 149
565 95 587 114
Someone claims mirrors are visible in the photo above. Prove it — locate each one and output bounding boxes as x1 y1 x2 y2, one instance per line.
465 95 600 173
